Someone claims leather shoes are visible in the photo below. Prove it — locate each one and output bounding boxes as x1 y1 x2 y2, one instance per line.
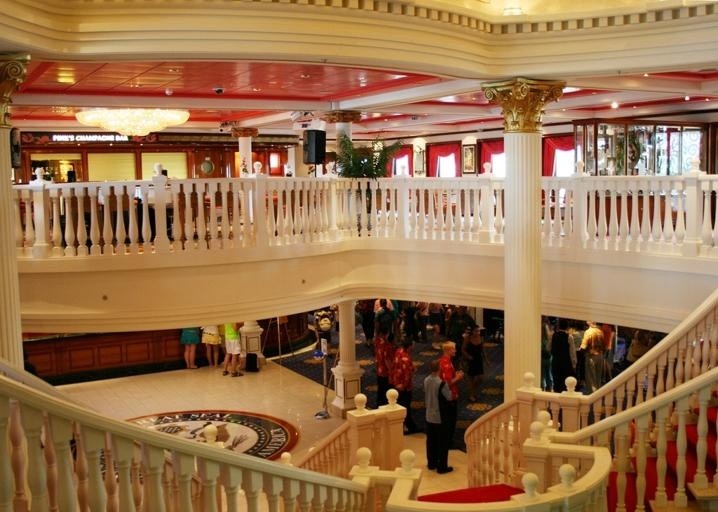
437 466 454 474
427 463 438 470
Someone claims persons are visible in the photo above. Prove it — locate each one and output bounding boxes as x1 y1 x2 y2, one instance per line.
223 322 244 377
200 325 221 369
179 327 200 369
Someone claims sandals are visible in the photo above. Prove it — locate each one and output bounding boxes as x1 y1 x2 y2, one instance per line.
468 395 481 402
231 371 244 377
222 370 229 376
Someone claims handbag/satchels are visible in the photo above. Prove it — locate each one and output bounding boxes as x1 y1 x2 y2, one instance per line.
437 380 456 423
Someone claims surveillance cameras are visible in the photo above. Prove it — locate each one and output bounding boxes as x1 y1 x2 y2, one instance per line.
215 88 224 95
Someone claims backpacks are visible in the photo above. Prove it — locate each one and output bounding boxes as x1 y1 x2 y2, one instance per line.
245 352 259 372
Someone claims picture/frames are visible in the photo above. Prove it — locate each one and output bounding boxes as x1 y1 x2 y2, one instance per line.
463 144 477 173
415 150 424 174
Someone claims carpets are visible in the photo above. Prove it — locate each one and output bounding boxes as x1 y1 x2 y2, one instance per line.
272 314 677 455
99 410 299 489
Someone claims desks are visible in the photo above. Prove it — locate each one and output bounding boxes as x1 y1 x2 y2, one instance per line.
588 195 666 237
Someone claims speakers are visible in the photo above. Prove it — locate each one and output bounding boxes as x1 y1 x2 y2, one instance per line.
303 130 326 164
